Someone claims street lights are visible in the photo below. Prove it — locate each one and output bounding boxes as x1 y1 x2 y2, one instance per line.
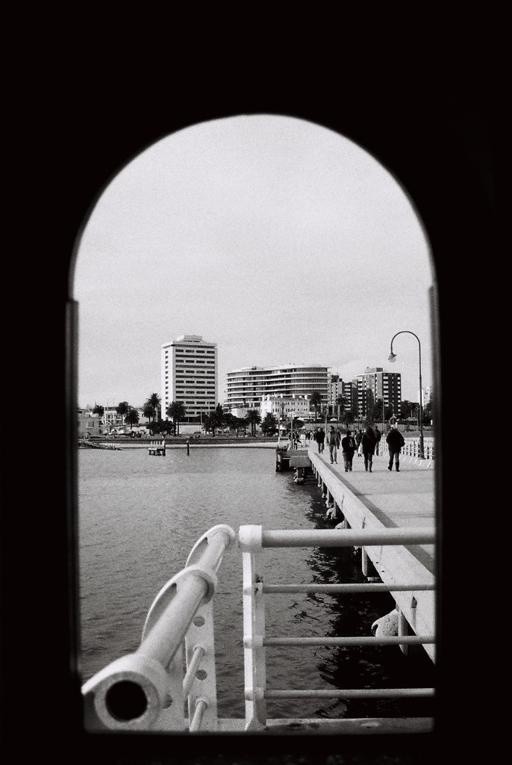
387 331 424 458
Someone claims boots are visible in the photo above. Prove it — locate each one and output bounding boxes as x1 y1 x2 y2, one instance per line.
345 460 401 472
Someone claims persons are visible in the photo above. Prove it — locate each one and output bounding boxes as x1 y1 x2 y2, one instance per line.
305 425 381 473
386 424 405 473
289 430 302 451
